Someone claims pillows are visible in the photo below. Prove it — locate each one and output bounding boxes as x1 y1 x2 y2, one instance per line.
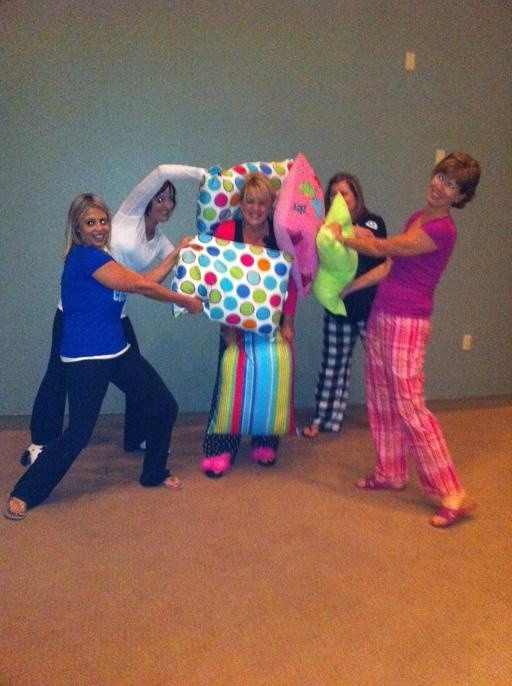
172 233 293 342
312 190 358 318
195 158 295 233
273 152 326 298
206 325 296 435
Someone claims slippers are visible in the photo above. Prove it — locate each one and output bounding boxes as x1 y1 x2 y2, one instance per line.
164 475 184 489
432 495 474 527
357 476 404 492
4 496 26 520
302 424 319 438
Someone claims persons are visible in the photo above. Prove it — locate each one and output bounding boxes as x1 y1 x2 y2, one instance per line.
18 162 228 468
3 192 206 522
322 148 482 529
199 169 299 479
301 169 389 439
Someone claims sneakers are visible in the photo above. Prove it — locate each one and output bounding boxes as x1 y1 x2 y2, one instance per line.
20 443 43 467
252 446 276 466
200 453 230 478
